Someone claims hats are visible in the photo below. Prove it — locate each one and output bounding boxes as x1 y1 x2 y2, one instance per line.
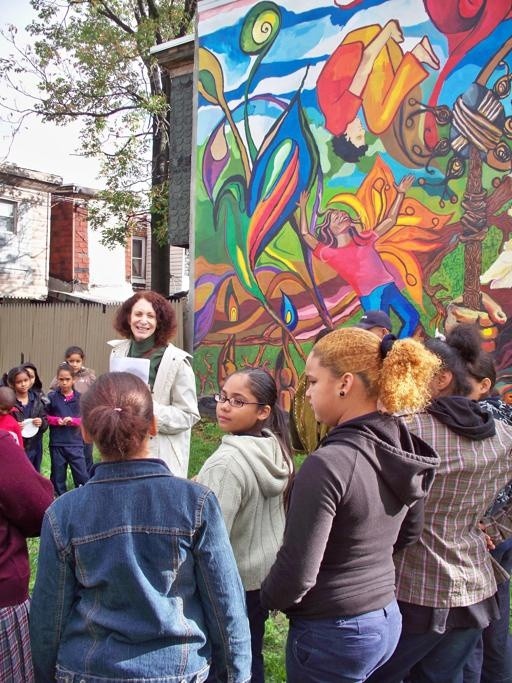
352 309 392 332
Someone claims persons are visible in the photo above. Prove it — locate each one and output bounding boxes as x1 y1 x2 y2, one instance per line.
29 371 254 683
45 361 90 496
315 17 441 163
475 355 512 683
258 326 443 683
0 428 56 683
362 323 512 683
0 386 24 450
2 366 49 464
22 361 47 474
441 238 512 354
48 346 96 473
295 175 420 340
190 365 297 683
287 327 336 457
105 290 202 480
356 309 394 341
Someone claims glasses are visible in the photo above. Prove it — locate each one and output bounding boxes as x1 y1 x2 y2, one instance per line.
215 393 268 408
